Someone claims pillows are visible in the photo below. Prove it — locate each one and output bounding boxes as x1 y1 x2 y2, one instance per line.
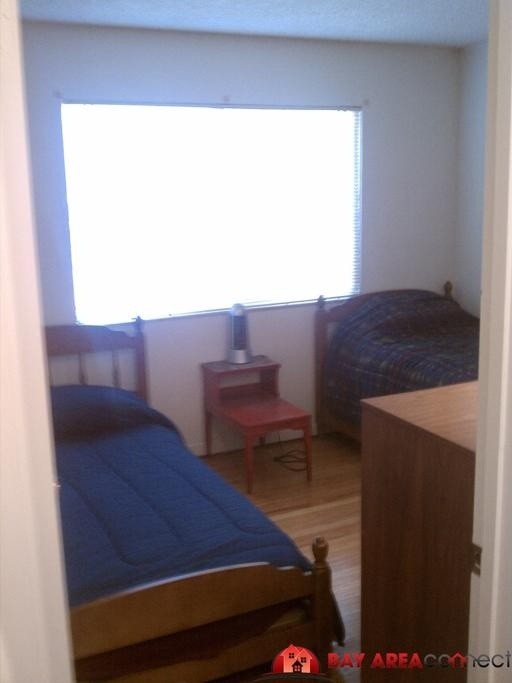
50 384 184 443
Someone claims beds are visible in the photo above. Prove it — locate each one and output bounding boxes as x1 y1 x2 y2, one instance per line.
315 279 480 445
46 315 346 683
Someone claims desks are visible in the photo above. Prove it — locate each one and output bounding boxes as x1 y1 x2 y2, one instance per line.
358 380 480 683
200 354 312 494
